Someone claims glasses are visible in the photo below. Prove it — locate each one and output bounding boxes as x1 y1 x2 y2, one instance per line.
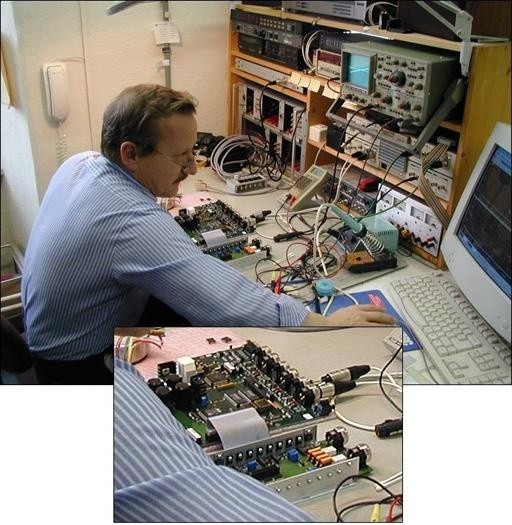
156 148 200 168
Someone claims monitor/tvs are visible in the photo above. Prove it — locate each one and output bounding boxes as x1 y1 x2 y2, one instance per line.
438 122 511 343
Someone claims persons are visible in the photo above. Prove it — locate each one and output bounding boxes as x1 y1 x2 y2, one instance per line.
21 82 399 384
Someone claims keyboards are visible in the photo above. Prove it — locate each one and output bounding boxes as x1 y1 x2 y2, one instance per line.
389 267 512 385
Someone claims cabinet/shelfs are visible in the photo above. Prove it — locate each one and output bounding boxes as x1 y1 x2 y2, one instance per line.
227 0 511 271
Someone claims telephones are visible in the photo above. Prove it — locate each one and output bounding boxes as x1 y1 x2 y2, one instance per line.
42 61 69 127
